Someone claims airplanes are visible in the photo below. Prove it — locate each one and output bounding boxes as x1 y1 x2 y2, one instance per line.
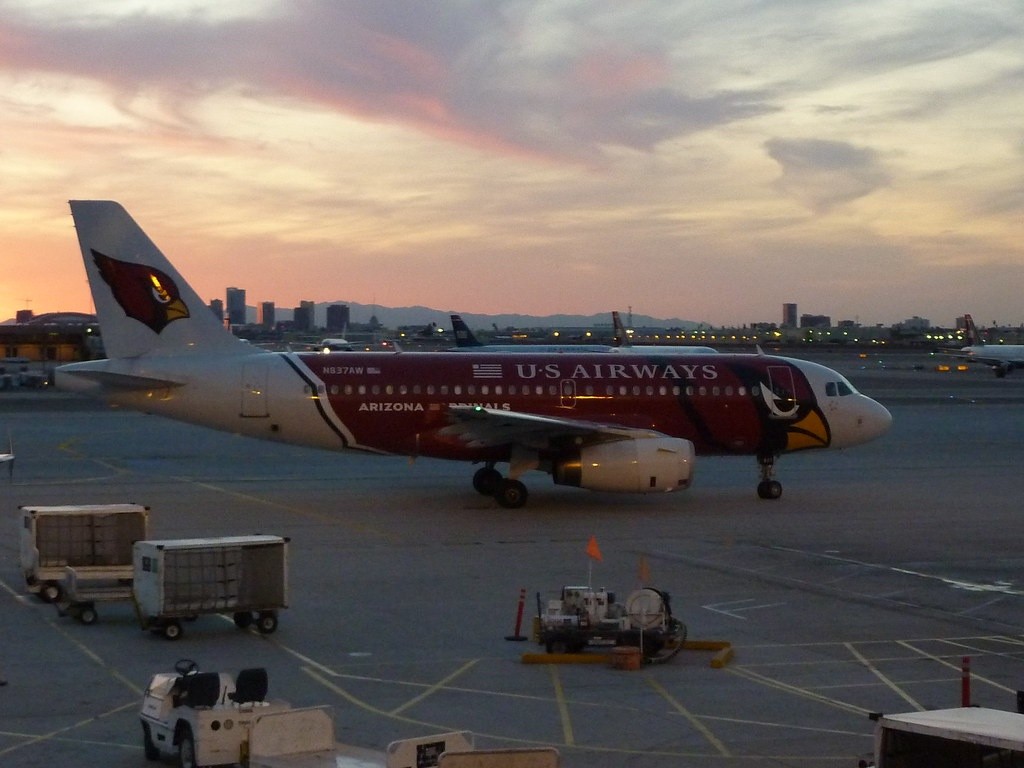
941 313 1024 378
51 198 897 507
